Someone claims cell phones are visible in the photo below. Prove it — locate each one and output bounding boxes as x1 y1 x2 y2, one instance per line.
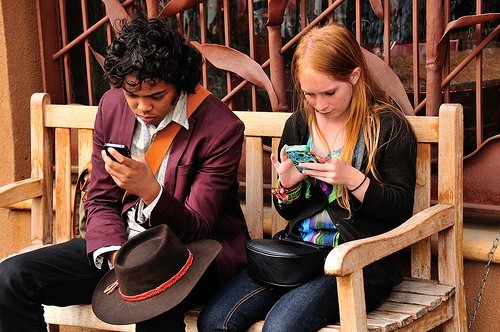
104 143 131 165
286 145 320 173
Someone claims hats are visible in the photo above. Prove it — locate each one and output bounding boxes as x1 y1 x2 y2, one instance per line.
91 223 223 325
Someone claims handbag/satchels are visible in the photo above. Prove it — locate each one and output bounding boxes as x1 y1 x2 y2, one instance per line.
244 228 335 288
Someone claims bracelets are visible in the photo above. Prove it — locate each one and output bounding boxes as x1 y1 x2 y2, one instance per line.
349 175 367 192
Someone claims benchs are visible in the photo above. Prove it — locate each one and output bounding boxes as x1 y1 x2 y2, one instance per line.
1 93 468 331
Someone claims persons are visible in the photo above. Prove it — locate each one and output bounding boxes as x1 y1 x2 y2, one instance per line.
197 23 418 332
0 11 251 332
